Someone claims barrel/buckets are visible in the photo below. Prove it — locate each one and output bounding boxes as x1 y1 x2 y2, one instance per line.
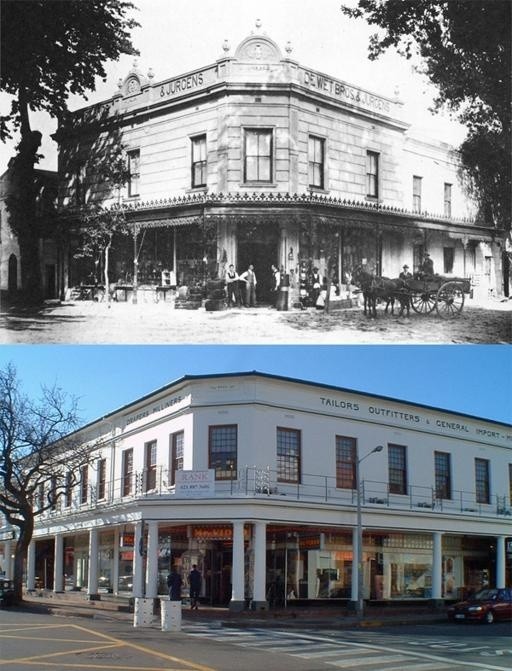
134 597 154 628
160 599 181 632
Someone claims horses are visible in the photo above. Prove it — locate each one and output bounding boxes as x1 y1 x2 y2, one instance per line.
350 264 411 319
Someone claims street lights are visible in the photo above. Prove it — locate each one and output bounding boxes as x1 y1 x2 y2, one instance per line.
354 444 383 619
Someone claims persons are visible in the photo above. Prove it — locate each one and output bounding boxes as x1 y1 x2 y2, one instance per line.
167 564 184 603
289 265 361 312
417 252 434 280
398 265 412 280
239 264 260 307
186 564 203 610
268 263 280 308
224 263 241 308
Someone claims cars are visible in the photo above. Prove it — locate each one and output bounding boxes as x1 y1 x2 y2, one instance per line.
446 587 512 624
1 566 17 610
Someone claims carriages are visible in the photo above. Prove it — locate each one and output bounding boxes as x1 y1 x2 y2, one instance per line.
349 261 471 320
